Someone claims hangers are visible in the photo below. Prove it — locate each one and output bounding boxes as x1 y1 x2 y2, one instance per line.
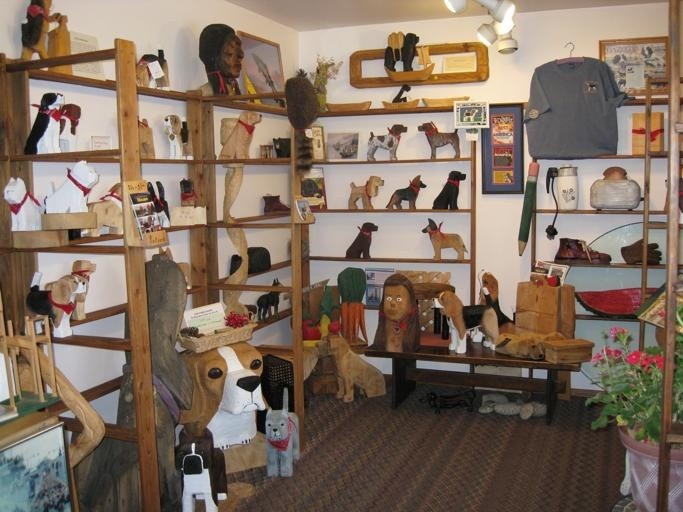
557 42 584 64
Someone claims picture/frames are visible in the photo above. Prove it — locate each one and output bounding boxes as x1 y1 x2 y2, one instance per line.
599 36 669 95
482 104 524 194
236 30 288 109
0 420 74 512
453 100 490 129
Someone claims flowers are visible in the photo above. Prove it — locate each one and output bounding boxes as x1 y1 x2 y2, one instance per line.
584 306 683 444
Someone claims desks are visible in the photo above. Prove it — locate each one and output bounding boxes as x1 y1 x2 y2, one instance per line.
364 333 581 424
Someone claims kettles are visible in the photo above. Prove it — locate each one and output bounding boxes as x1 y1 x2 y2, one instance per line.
543 164 580 208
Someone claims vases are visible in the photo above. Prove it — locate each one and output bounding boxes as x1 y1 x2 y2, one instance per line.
619 422 683 512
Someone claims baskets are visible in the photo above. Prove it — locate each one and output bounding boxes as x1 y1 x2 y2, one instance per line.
256 353 294 435
177 311 255 352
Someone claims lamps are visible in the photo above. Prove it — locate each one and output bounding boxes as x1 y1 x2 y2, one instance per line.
444 0 518 54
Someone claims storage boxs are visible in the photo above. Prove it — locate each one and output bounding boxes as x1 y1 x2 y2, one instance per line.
544 338 595 364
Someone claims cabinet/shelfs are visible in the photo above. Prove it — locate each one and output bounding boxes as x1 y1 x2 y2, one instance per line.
532 98 682 398
0 38 476 512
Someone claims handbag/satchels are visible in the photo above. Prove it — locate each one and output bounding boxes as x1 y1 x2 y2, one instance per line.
512 274 577 341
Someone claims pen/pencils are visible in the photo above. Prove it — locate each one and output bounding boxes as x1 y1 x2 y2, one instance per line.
517 156 540 257
